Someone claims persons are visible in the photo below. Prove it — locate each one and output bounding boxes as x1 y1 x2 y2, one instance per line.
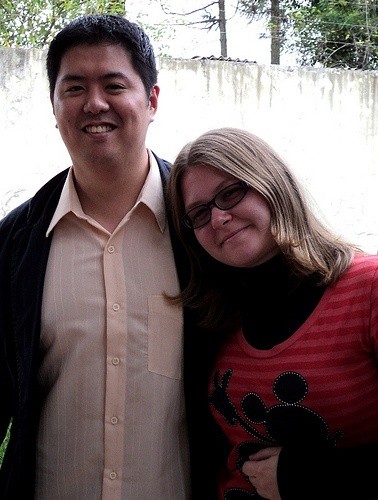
0 13 213 500
165 126 378 500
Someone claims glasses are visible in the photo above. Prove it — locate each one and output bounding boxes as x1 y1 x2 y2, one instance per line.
182 180 248 229
